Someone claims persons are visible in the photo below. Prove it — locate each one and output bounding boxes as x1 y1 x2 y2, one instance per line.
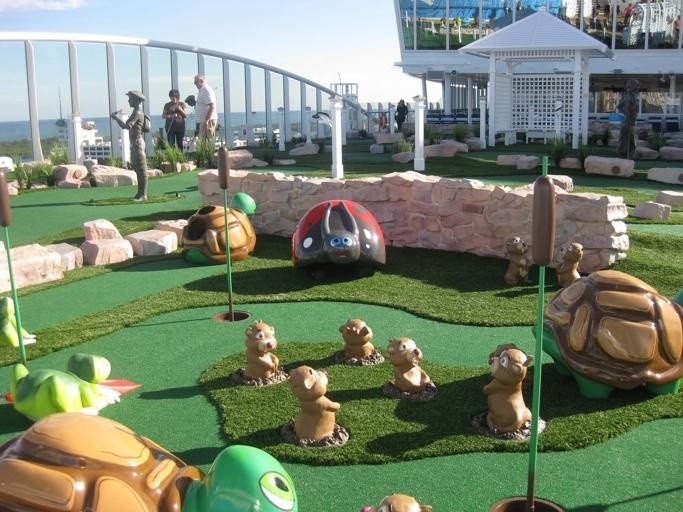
396 100 408 130
616 78 640 161
416 0 680 43
161 89 189 153
193 73 219 168
380 111 388 129
111 89 150 203
